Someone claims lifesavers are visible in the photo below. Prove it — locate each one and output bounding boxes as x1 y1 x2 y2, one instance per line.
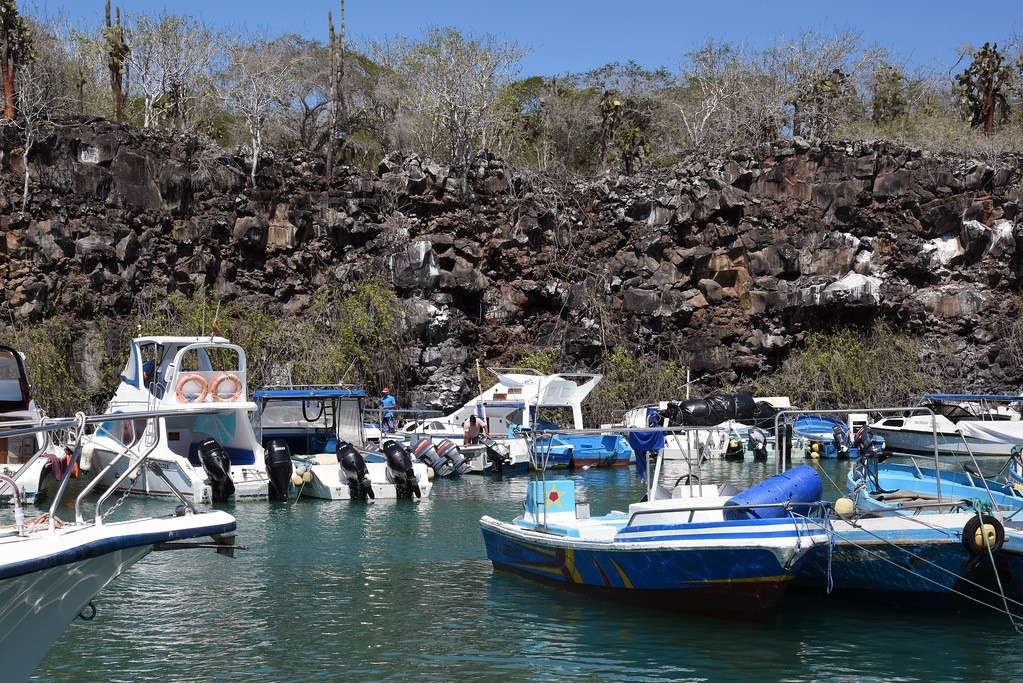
854 426 866 441
210 373 243 403
175 373 209 404
960 514 1006 559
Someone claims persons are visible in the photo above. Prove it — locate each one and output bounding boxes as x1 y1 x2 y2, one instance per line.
463 415 492 448
378 389 397 433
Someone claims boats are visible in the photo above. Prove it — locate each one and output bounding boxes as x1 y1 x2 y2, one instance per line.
250 390 434 501
368 361 1023 470
0 403 251 683
477 426 836 633
0 345 67 506
776 407 1006 604
72 336 299 504
845 429 1023 591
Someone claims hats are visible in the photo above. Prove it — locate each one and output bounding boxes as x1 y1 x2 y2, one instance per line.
382 388 390 394
